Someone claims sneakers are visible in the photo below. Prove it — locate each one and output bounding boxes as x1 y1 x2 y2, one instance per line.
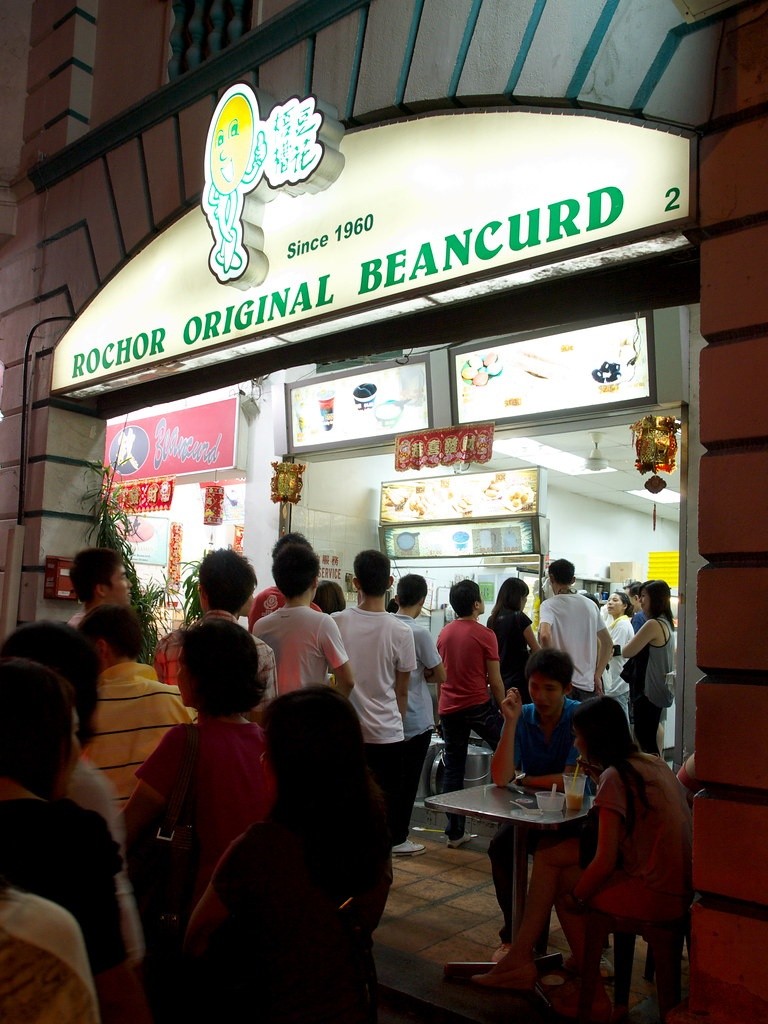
390 839 426 857
491 944 510 964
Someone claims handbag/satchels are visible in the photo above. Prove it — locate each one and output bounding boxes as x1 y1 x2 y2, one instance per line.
138 723 196 928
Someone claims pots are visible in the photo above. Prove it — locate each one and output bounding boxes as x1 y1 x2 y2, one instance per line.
372 399 403 429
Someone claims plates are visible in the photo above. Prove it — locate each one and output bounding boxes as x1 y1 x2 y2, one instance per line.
460 354 502 384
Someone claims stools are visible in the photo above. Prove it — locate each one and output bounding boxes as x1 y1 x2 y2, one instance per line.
580 912 690 1024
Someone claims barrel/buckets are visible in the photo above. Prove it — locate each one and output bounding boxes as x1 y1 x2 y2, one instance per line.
413 733 445 802
446 742 494 792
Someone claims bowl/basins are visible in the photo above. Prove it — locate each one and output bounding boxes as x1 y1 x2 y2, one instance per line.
535 791 566 811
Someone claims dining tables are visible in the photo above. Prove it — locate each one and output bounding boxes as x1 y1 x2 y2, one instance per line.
424 784 596 1018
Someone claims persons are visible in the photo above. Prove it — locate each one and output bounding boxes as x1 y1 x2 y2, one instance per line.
67 548 135 636
486 577 539 703
0 537 346 1024
470 697 693 991
185 686 389 1024
491 648 616 980
327 550 417 757
251 544 355 702
622 580 673 759
437 579 508 849
110 620 281 935
539 559 614 701
602 592 635 749
386 574 446 857
147 549 286 718
60 604 201 812
539 558 675 763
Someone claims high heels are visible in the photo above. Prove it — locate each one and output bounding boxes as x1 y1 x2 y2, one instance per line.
472 959 537 991
552 991 612 1022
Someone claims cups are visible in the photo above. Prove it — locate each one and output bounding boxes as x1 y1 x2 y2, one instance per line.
594 585 609 601
316 389 336 431
563 772 588 810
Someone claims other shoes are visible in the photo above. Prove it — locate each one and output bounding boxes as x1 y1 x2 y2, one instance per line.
447 832 470 849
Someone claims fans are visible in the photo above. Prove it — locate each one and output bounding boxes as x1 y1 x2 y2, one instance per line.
585 432 610 470
